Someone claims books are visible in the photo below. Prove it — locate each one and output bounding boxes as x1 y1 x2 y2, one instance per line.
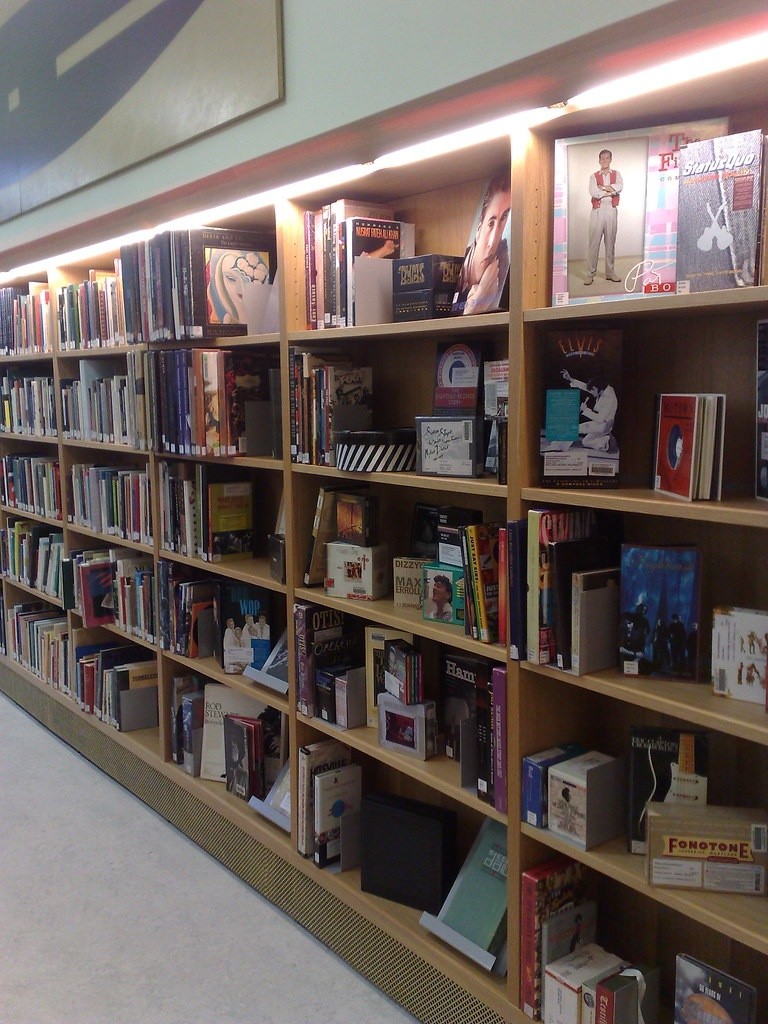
1 115 768 1023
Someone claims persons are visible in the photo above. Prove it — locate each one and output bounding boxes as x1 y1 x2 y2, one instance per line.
618 600 701 679
579 148 622 285
224 612 270 673
344 562 359 581
561 369 618 455
352 231 400 301
335 384 372 406
446 167 511 316
429 574 453 621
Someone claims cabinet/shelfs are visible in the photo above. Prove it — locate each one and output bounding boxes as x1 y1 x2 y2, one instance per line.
0 0 768 1024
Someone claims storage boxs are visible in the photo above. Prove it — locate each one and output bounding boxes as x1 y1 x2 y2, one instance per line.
392 253 464 323
626 722 711 856
335 675 349 729
315 659 363 724
268 533 286 584
548 751 617 846
326 540 389 601
711 605 768 705
422 562 465 627
334 427 416 473
360 791 458 916
544 942 625 1024
522 742 591 830
413 415 485 479
645 801 768 898
